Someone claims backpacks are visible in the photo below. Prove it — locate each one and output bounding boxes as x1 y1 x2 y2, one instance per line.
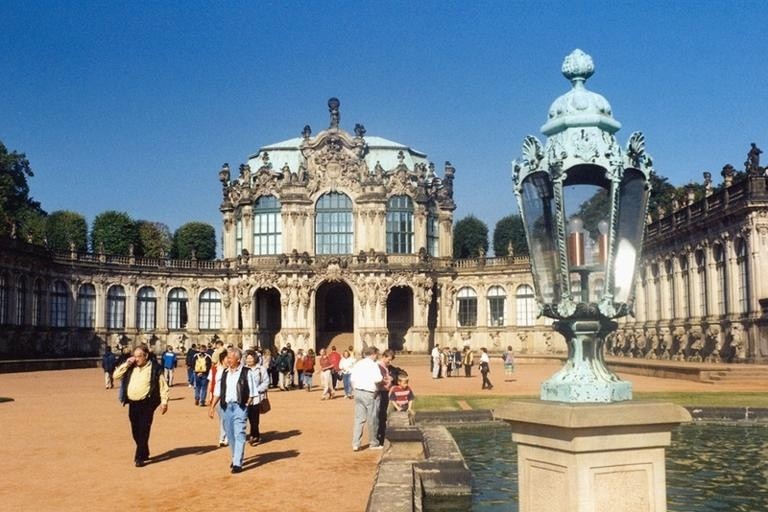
279 355 288 372
195 355 207 372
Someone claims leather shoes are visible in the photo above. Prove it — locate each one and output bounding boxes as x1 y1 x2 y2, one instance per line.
233 466 241 473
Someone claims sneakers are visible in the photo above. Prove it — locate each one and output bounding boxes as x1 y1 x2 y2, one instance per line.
136 456 144 467
353 446 358 451
369 445 384 448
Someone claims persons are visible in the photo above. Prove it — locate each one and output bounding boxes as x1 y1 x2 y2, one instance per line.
209 348 255 473
112 345 169 467
504 346 514 381
432 344 473 378
205 352 228 446
388 374 414 413
479 348 493 390
350 347 383 451
244 351 270 446
375 349 395 445
102 346 115 389
114 341 365 406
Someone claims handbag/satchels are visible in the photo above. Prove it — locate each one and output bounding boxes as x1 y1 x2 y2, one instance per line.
259 398 270 413
481 367 488 373
